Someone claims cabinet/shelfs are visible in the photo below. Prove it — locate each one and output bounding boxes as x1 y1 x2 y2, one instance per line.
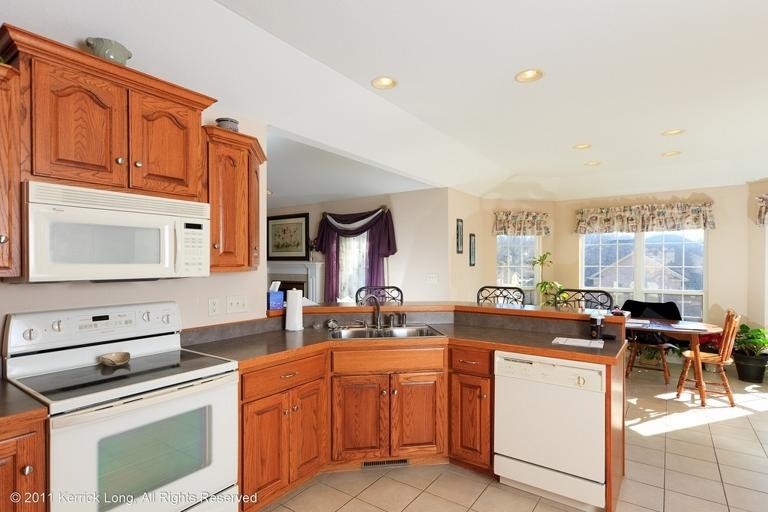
0 63 21 280
184 331 328 512
0 377 48 512
450 335 493 480
1 20 218 204
202 126 266 273
331 341 449 470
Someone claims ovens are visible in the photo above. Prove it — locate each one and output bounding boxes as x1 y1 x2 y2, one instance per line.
47 371 243 512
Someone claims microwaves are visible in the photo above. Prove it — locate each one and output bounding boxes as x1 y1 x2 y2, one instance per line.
29 180 210 282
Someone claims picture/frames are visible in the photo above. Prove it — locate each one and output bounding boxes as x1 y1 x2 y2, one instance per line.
456 219 463 254
264 212 311 261
469 233 479 267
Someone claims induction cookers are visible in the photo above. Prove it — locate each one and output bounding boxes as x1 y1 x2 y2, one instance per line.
11 349 238 414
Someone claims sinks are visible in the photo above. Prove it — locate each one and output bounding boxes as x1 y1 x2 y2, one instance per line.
328 324 448 340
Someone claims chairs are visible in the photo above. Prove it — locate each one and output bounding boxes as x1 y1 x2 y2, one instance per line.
554 288 613 309
676 308 742 407
476 285 525 304
355 286 405 304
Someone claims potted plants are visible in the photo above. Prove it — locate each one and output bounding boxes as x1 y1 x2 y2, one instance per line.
625 302 676 385
731 324 768 384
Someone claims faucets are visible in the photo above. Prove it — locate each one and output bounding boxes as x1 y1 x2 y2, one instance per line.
359 293 383 328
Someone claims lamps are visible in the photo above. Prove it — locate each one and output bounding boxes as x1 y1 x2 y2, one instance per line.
371 76 397 90
514 68 543 83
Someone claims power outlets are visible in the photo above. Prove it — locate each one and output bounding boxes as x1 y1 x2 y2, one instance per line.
226 296 249 312
209 297 225 316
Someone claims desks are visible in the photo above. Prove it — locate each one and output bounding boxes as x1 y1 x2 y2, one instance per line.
623 317 724 391
